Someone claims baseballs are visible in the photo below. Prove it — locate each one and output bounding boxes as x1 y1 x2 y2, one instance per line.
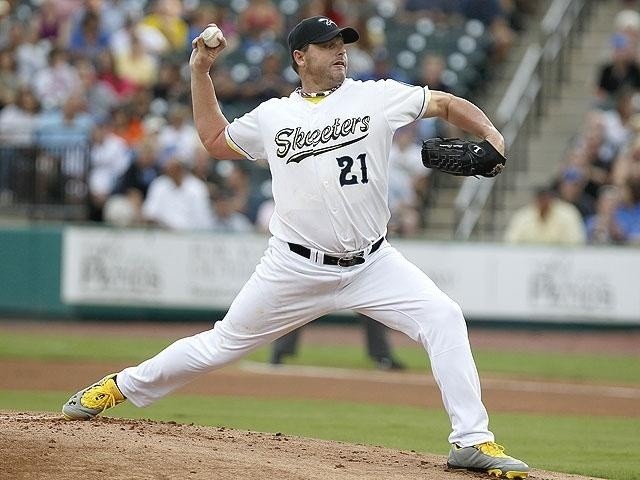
202 27 223 48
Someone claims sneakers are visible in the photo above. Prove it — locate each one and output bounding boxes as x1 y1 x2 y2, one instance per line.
446 441 530 479
58 371 124 419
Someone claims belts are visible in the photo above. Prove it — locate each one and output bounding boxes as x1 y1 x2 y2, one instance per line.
287 236 386 269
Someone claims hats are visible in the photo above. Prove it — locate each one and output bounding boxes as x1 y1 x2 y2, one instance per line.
286 14 360 51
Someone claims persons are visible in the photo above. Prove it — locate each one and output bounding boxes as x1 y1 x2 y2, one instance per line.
0 0 526 245
267 312 407 369
61 17 531 479
505 1 640 245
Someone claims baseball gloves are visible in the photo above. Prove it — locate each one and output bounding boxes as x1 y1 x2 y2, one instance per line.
421 137 507 178
